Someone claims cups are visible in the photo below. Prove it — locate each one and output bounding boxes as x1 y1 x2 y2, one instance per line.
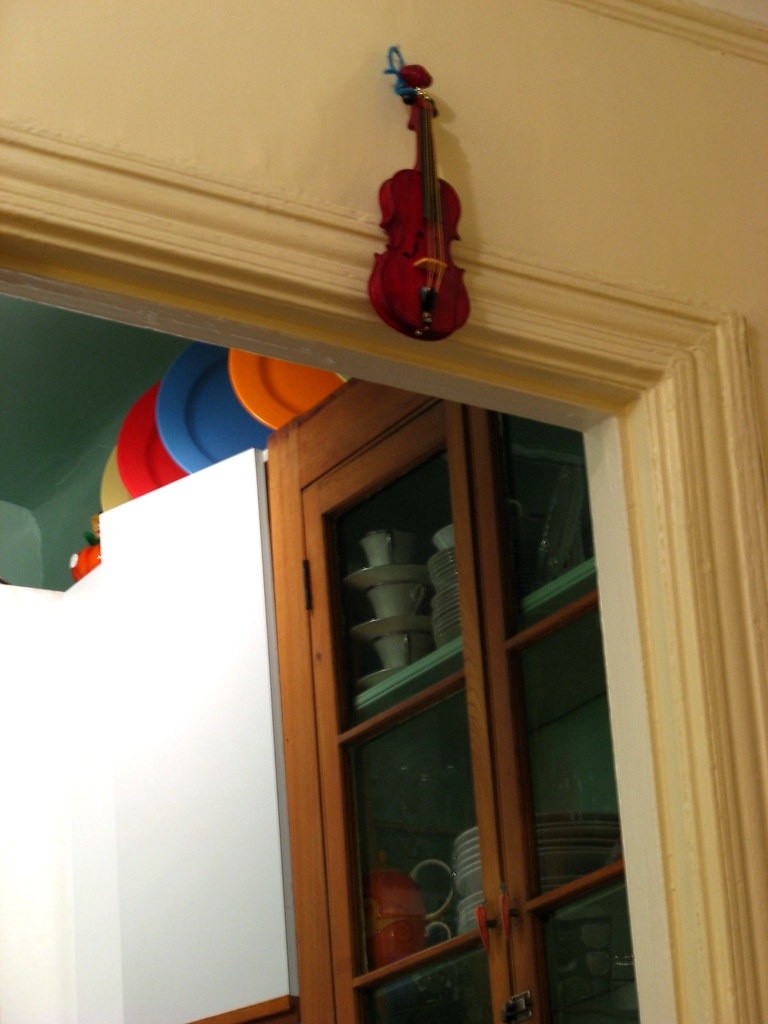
373 631 428 669
432 524 455 550
366 581 426 619
362 530 417 566
366 918 452 971
364 860 454 920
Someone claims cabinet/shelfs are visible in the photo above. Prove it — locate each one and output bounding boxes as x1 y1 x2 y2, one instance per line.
267 376 638 1022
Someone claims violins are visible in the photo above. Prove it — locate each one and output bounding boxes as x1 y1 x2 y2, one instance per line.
367 63 472 344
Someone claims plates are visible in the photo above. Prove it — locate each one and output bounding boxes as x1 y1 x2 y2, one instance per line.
99 444 132 512
358 670 401 689
344 564 426 590
351 615 430 643
229 348 346 431
156 341 274 475
427 550 462 647
118 383 190 498
452 811 622 935
